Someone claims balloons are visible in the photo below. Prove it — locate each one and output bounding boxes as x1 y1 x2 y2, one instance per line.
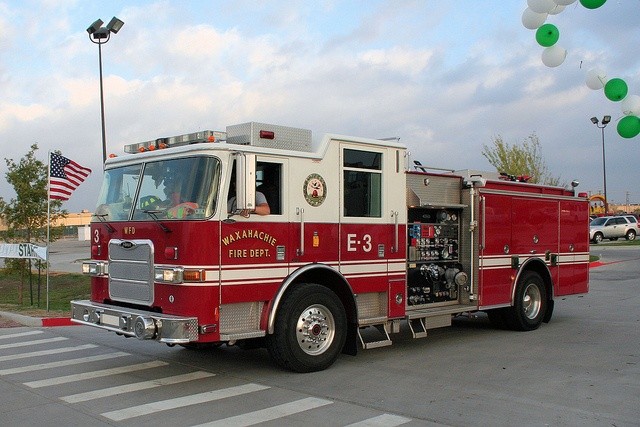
622 95 640 115
536 23 559 47
604 78 627 102
554 0 576 6
586 67 607 91
526 1 555 14
617 115 640 138
541 47 568 67
520 7 547 30
579 0 608 10
550 5 563 14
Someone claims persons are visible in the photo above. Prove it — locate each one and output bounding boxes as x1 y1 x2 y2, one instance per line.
227 177 271 215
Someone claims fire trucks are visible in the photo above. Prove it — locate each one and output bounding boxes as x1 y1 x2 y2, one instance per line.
70 122 590 374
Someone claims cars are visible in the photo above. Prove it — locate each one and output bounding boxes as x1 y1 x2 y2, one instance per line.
589 215 640 244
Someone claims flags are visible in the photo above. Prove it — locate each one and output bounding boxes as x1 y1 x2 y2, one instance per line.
50 152 93 200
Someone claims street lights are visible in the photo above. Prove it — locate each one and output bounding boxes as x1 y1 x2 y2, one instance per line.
590 116 611 214
86 16 124 165
